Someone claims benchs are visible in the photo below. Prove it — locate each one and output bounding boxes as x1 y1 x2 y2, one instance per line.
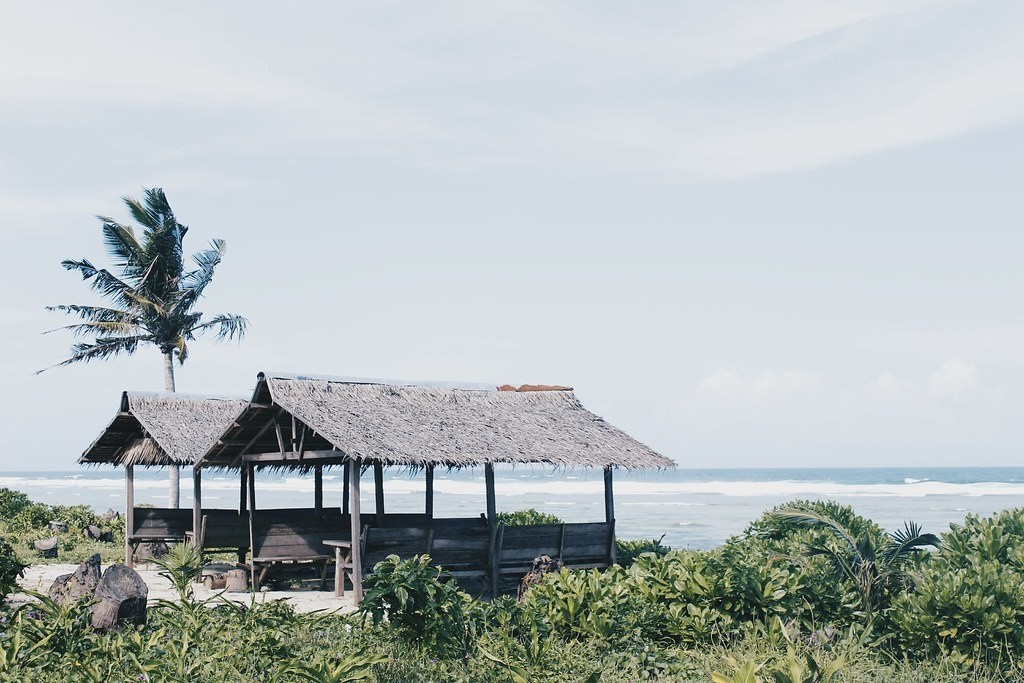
246 507 341 514
129 508 238 570
249 514 432 591
497 519 616 599
322 525 495 599
200 515 249 565
